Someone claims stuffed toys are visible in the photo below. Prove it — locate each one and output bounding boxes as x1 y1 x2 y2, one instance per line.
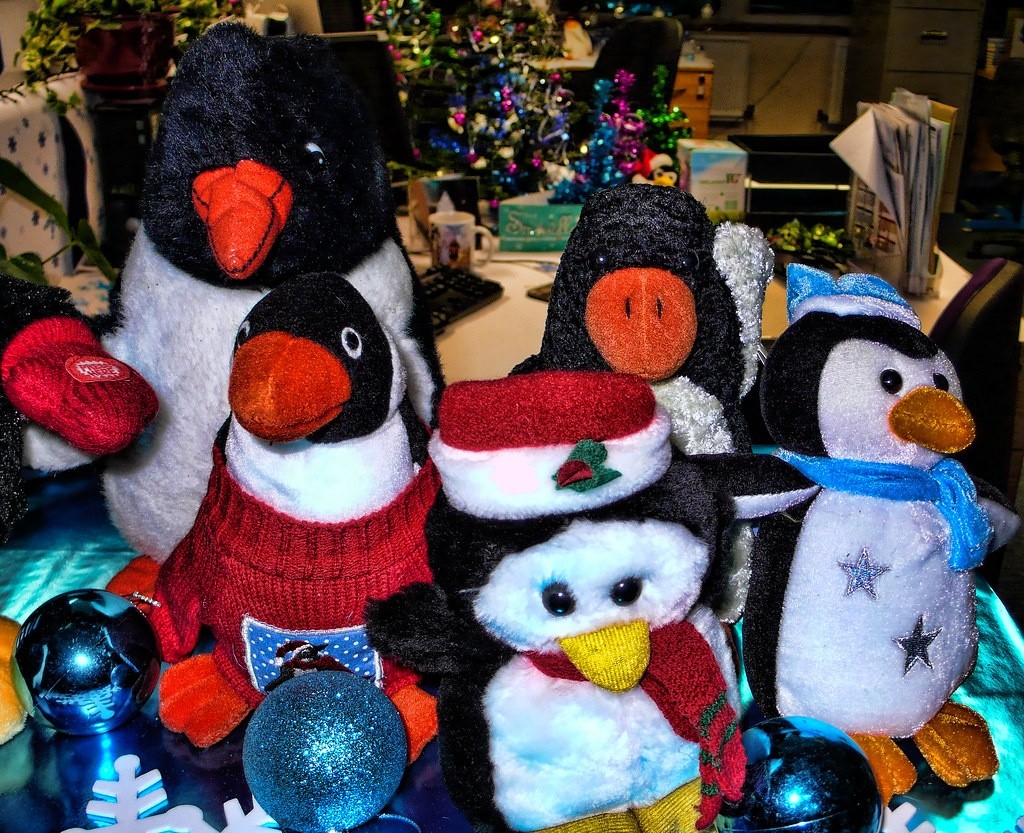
510 183 768 538
359 372 750 833
136 274 440 778
23 22 444 600
0 273 159 537
745 265 1024 802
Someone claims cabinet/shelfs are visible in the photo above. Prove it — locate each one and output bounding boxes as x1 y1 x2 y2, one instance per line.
668 50 715 139
841 0 981 213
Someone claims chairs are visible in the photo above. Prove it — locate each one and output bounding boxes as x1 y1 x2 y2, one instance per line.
929 257 1024 495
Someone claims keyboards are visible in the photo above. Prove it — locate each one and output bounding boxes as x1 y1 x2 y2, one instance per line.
417 264 503 335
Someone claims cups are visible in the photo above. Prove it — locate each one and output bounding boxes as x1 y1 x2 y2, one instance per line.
429 210 494 270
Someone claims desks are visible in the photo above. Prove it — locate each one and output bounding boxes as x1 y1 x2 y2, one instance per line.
410 237 973 385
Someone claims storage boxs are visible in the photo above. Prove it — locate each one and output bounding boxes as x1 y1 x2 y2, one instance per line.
728 133 853 212
677 139 748 221
